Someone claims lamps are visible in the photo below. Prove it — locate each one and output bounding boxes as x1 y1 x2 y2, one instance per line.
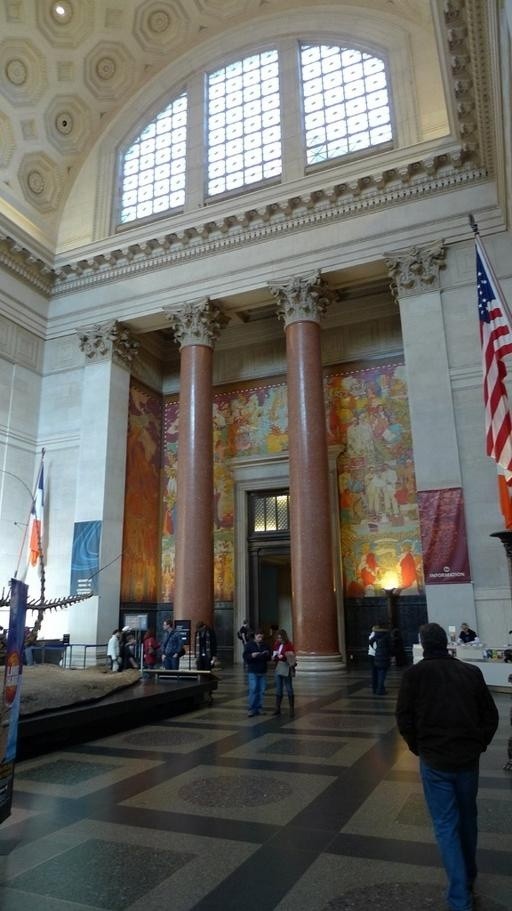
378 570 406 666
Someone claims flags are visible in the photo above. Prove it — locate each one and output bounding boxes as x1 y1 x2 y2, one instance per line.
468 210 512 493
30 461 45 564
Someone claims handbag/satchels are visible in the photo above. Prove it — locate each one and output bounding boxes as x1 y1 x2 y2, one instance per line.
276 660 289 676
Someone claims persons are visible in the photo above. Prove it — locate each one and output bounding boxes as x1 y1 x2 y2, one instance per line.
395 621 500 910
104 605 299 721
397 540 417 589
370 620 393 695
368 624 379 694
360 553 379 587
457 623 479 645
168 377 405 520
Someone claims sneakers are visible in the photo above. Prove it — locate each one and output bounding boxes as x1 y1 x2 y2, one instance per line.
248 709 265 716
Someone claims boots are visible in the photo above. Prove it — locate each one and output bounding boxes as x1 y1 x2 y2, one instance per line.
288 694 294 717
272 694 283 715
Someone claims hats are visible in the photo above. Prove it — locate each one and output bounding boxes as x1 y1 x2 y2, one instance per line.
422 623 447 650
